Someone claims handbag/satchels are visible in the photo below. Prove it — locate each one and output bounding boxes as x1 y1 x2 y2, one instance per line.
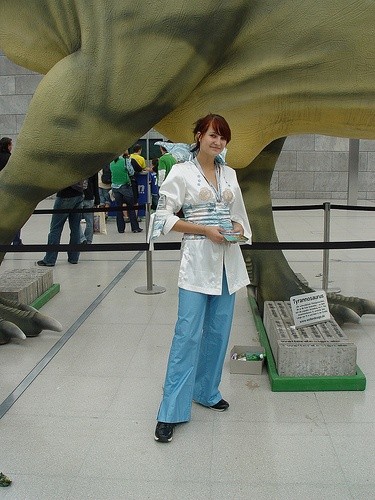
124 179 139 205
93 205 107 236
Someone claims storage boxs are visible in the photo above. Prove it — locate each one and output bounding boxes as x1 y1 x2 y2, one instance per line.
229 345 265 375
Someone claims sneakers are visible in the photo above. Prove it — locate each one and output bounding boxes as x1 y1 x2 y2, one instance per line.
209 399 230 411
154 422 176 442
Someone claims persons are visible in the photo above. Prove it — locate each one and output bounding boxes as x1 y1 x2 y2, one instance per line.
151 113 252 442
0 137 176 267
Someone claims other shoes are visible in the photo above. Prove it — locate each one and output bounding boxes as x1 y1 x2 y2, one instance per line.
68 259 77 264
132 228 143 233
37 261 54 266
119 230 124 233
